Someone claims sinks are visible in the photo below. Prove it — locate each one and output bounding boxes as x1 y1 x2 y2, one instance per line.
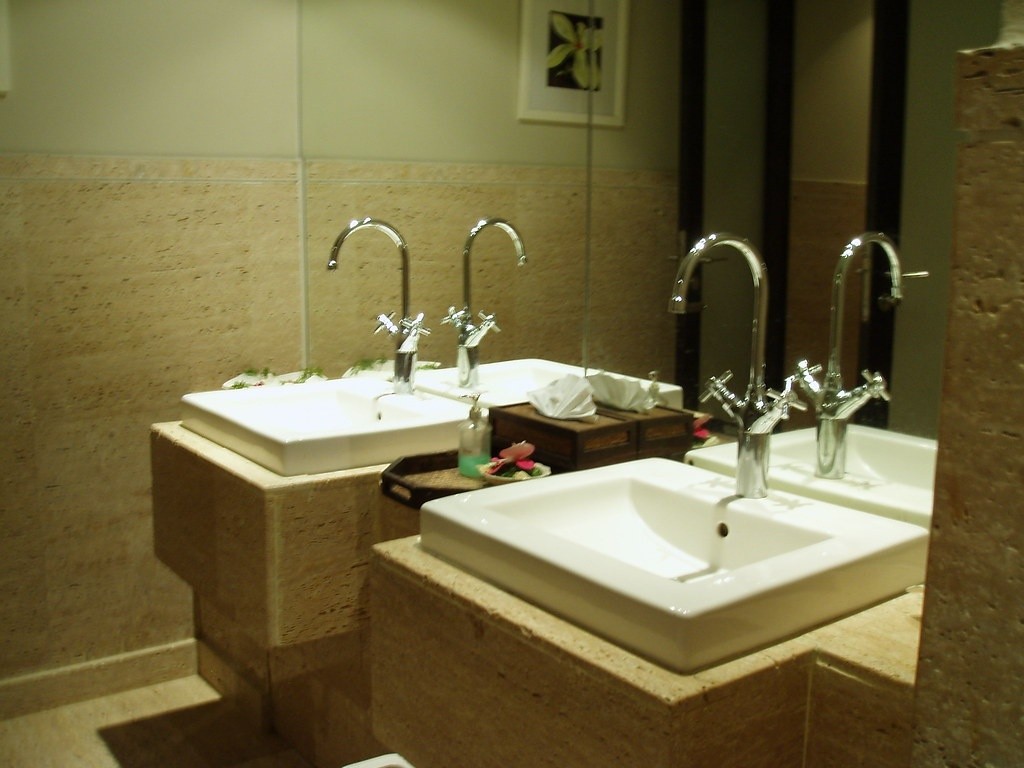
412 359 682 412
421 456 928 674
689 423 938 530
182 374 490 475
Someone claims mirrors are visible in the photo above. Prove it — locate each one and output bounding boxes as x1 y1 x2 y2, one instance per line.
294 1 1002 528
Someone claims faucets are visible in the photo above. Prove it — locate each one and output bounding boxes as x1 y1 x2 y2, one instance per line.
816 235 901 480
328 219 410 379
459 218 529 372
668 236 772 496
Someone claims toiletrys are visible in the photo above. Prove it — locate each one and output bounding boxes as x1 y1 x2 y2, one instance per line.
455 391 492 479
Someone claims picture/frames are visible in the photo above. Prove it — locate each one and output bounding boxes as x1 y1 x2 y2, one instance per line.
517 0 632 128
